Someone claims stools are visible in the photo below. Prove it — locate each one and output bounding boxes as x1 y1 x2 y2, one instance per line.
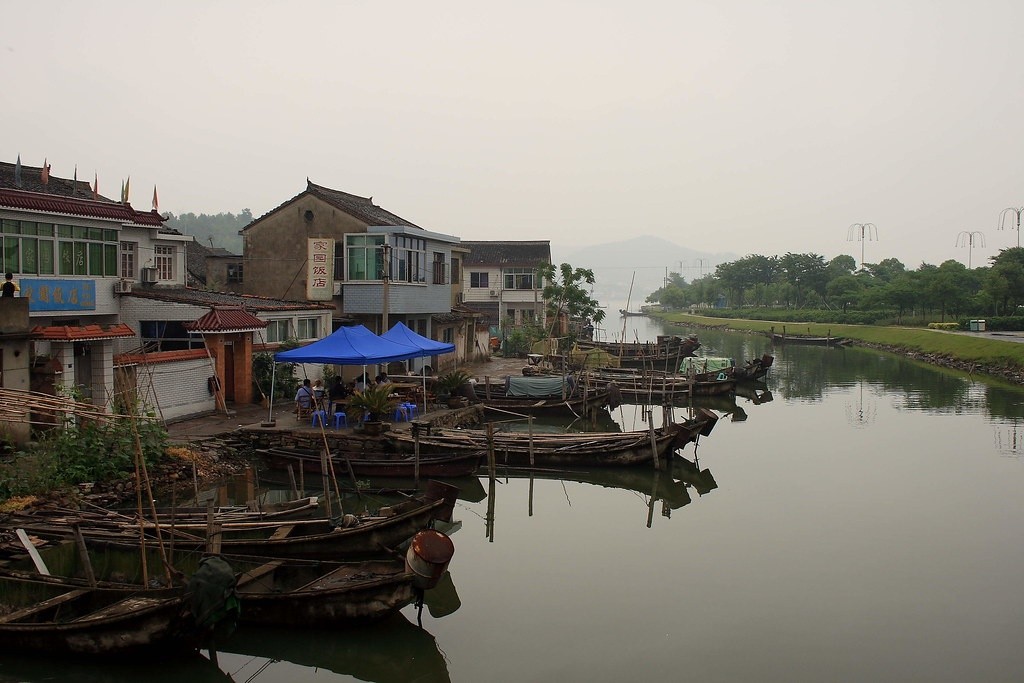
313 411 327 428
333 412 348 430
390 402 419 423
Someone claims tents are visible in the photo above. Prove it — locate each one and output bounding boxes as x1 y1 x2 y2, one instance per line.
363 321 456 397
268 325 427 422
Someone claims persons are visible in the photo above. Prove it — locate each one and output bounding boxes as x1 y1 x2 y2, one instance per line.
312 380 325 391
327 371 390 412
469 378 479 388
0 272 20 297
291 378 312 414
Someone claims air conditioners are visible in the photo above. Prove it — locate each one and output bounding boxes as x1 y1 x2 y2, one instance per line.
489 289 498 296
115 281 131 293
143 268 159 283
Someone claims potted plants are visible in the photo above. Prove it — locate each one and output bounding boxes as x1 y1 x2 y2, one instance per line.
432 370 473 409
344 382 399 436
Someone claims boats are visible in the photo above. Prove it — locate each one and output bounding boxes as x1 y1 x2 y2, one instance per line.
425 353 774 411
569 332 702 364
618 309 644 316
268 408 721 478
0 480 467 671
768 325 853 345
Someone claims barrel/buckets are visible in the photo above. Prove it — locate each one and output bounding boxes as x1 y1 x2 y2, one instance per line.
425 571 461 618
405 527 454 590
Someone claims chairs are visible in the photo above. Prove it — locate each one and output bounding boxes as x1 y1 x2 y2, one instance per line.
408 385 450 412
295 390 326 423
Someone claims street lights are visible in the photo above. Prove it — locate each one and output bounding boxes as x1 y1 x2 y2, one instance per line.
954 231 987 270
998 207 1024 248
846 223 879 271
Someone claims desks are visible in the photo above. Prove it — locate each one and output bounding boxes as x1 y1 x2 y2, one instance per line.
332 382 420 423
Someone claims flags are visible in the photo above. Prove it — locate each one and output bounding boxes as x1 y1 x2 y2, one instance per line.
120 184 124 201
73 169 78 190
125 181 129 201
41 162 49 185
94 179 98 200
14 159 22 188
153 188 159 208
